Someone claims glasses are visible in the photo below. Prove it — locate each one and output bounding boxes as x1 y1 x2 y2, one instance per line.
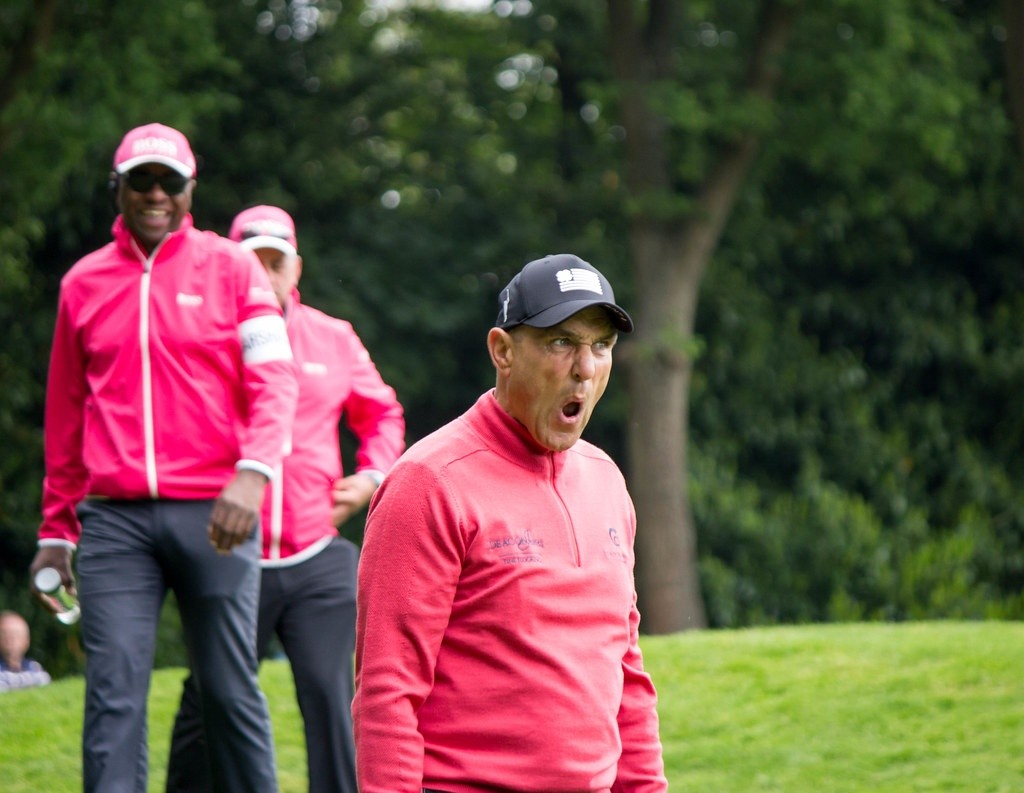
126 168 188 196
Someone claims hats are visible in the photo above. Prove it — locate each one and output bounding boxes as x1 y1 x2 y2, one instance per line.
113 123 196 178
229 205 297 257
495 254 634 334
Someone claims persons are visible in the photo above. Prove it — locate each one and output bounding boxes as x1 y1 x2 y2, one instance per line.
167 205 406 793
0 612 52 692
29 122 298 793
349 253 668 793
